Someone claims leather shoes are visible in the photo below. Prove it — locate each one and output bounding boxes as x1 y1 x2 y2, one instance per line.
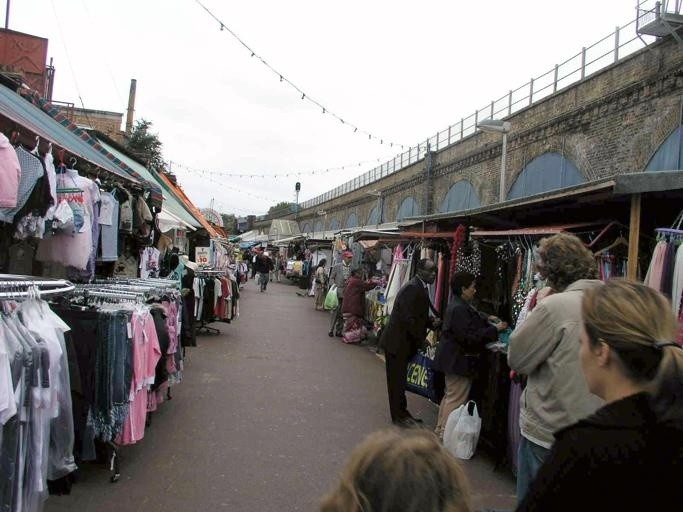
336 333 343 336
393 414 424 428
329 331 334 337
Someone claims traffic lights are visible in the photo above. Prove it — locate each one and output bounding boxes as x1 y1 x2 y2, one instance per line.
296 182 300 191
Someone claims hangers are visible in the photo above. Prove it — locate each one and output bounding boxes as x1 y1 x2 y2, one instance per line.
15 131 20 146
0 280 43 304
589 231 595 243
69 277 177 316
416 237 424 250
85 164 125 186
594 230 628 256
196 270 227 280
70 156 77 171
48 142 53 154
427 239 448 256
509 227 553 251
35 135 39 149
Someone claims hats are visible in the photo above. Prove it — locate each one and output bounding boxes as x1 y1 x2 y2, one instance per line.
343 252 353 258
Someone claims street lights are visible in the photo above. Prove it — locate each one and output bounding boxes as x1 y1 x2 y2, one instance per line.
477 119 511 203
316 211 327 230
367 189 383 225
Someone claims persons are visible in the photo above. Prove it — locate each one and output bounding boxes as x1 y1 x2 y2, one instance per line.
243 249 282 292
518 276 683 510
319 428 473 511
315 259 330 311
328 252 352 338
381 259 442 427
507 232 607 510
341 269 380 343
431 271 506 443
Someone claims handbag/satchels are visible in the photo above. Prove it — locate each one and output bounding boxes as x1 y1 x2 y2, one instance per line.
405 353 434 398
315 278 322 283
452 344 483 377
